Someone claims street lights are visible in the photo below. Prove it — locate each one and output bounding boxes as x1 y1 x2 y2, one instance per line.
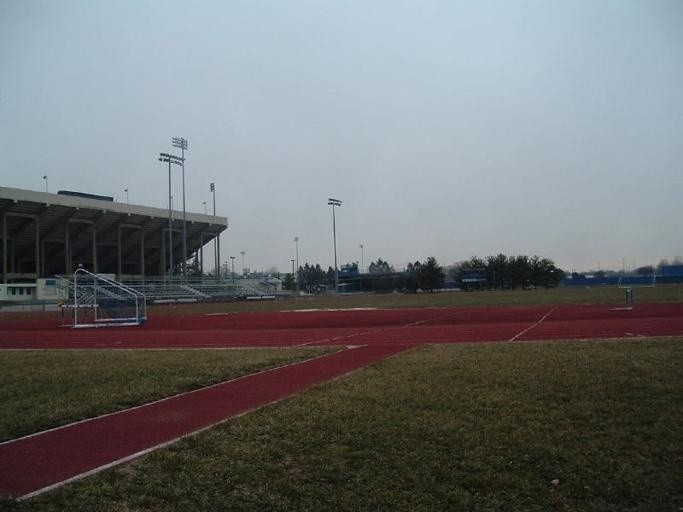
230 256 235 284
171 136 187 277
210 182 218 280
293 236 299 274
43 175 49 192
239 251 245 276
123 187 129 204
359 244 364 274
157 152 185 284
327 197 342 291
202 201 207 214
290 259 295 278
